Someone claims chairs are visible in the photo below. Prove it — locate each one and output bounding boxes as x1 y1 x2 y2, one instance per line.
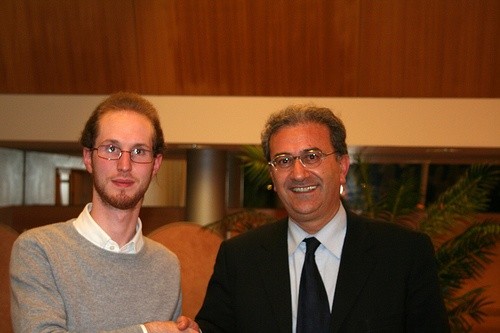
0 225 21 333
145 221 226 321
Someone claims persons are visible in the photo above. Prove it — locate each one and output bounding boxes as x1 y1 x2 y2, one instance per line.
9 93 197 333
176 103 449 333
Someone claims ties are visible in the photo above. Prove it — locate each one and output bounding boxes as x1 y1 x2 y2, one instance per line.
295 236 333 333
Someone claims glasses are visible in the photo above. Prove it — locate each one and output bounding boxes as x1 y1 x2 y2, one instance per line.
268 149 339 172
89 145 158 164
